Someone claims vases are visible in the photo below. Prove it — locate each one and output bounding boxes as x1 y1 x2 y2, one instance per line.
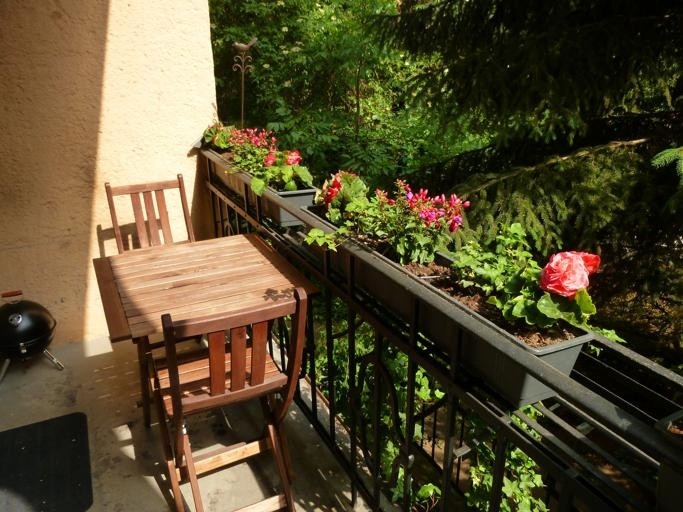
204 148 316 228
297 205 592 407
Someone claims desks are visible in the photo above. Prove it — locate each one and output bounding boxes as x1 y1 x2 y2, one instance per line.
107 232 310 465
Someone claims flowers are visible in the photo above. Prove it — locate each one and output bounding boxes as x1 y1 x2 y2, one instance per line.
315 170 602 329
205 116 314 194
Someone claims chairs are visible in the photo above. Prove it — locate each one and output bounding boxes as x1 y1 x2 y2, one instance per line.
104 177 249 408
152 287 309 512
